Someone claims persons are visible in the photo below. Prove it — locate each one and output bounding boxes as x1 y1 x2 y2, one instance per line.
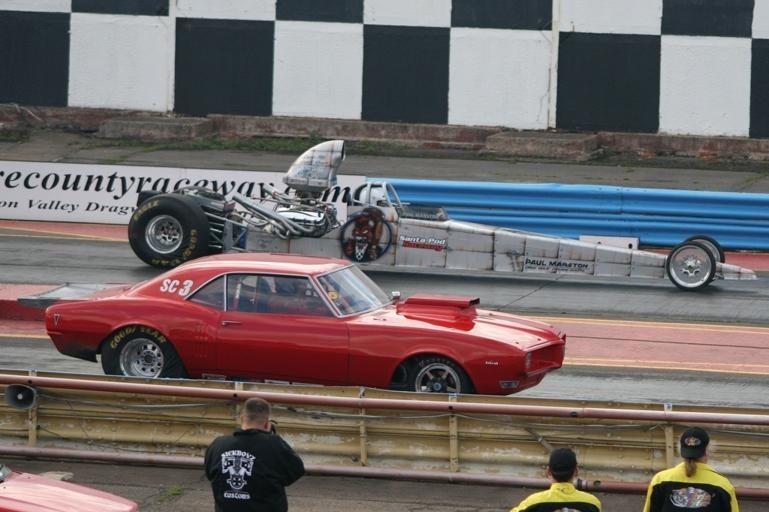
266 277 359 318
203 397 305 512
509 447 602 512
642 426 739 512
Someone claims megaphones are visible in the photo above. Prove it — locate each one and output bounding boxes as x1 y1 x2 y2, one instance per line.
6 384 37 412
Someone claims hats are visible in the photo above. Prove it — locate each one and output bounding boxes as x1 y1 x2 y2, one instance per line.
680 426 709 458
549 448 576 472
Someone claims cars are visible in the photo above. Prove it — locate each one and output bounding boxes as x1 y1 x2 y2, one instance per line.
44 252 566 395
0 464 139 512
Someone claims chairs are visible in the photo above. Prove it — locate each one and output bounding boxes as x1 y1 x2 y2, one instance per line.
255 276 271 310
